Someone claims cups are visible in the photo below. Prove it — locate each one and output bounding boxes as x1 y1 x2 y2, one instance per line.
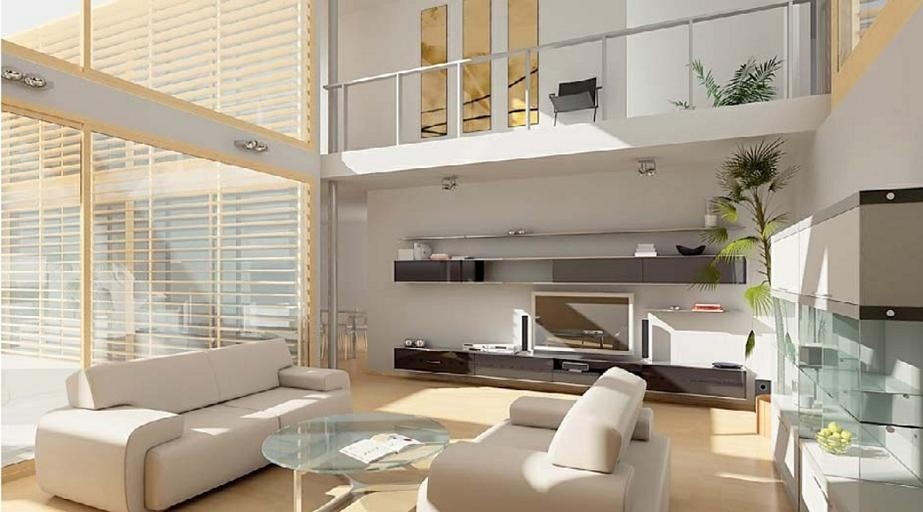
800 395 814 408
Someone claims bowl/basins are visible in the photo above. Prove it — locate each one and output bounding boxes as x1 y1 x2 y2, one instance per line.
815 429 857 454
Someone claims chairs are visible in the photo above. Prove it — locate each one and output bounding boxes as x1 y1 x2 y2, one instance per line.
320 310 368 361
549 77 602 127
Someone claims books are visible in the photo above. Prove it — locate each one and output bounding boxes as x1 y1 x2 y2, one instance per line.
691 303 725 312
338 432 425 465
634 243 658 257
430 253 475 260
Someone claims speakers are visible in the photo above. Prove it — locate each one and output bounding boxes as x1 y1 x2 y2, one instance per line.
644 256 747 283
461 261 484 282
521 315 528 351
393 260 461 282
642 318 649 358
551 258 643 283
754 379 772 412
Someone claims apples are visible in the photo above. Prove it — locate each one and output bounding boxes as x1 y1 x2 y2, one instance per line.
817 422 853 453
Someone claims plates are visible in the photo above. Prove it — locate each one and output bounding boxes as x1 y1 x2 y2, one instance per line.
712 362 743 369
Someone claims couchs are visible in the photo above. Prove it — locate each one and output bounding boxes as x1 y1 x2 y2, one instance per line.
35 339 354 512
416 366 670 512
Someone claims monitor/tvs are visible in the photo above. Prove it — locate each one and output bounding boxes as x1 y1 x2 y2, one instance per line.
529 290 636 360
